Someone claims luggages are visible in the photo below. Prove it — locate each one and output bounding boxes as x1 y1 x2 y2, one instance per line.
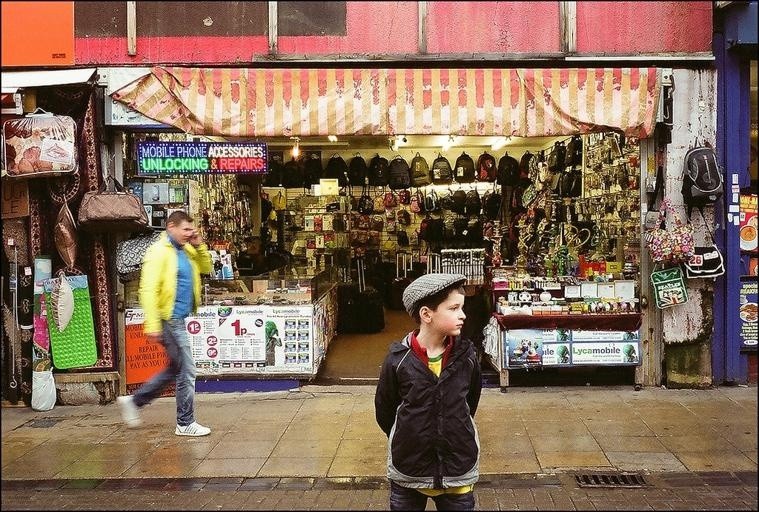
337 253 414 333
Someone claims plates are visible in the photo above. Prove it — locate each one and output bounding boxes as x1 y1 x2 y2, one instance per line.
740 215 759 251
739 302 759 323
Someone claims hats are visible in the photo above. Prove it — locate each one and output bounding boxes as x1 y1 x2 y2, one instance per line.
403 273 467 317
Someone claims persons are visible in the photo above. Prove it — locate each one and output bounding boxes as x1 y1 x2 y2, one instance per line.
375 273 483 512
116 211 212 436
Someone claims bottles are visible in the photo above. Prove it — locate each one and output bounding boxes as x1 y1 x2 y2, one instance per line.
152 184 159 200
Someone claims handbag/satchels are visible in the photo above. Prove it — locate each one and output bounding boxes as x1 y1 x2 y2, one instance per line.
2 106 77 183
650 267 689 310
75 178 149 235
647 198 695 264
681 244 725 280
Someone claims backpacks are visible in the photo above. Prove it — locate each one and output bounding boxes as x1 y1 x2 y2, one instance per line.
547 135 583 196
389 155 411 188
519 149 538 186
411 152 431 187
263 152 388 185
454 151 475 182
497 152 519 185
432 153 453 184
261 187 528 258
475 151 496 181
681 137 724 244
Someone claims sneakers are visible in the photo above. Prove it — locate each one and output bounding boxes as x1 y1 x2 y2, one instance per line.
117 395 139 429
175 422 211 437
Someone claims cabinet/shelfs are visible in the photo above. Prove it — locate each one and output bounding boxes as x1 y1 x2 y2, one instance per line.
483 259 649 393
194 254 340 382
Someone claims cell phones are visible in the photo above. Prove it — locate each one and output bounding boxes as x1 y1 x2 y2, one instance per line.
191 233 197 239
